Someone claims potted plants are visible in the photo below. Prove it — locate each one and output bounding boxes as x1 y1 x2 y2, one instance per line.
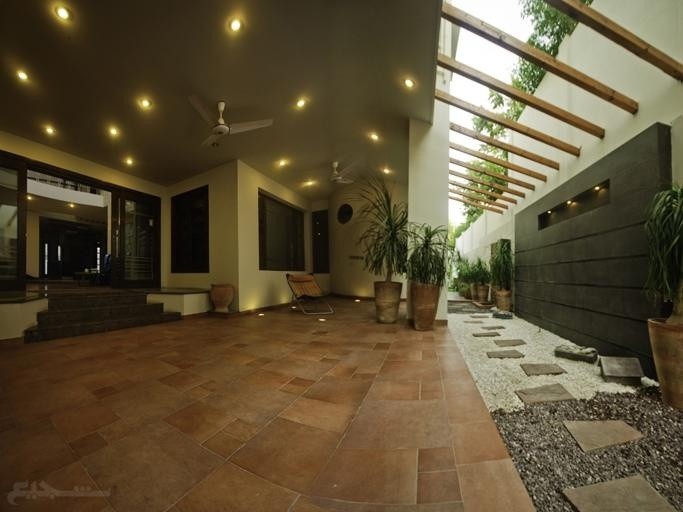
490 239 513 312
473 257 491 300
463 269 479 299
342 164 415 323
408 223 450 331
641 185 683 410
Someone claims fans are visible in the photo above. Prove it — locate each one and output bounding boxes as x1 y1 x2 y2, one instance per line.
187 94 273 147
313 160 357 189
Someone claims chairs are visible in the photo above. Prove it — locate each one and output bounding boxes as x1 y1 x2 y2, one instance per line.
286 273 334 315
